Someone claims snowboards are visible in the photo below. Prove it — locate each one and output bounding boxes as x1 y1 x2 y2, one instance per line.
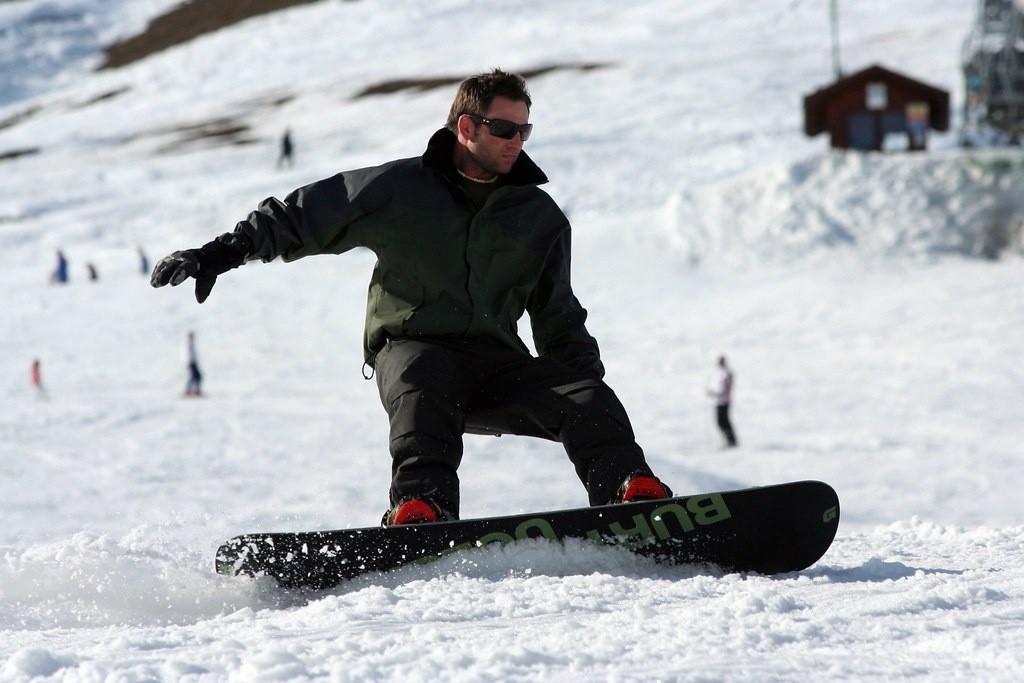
213 481 842 594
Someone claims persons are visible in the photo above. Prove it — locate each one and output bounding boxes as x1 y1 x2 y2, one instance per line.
151 66 675 530
276 128 292 167
137 247 147 274
86 264 97 280
29 360 41 389
55 251 67 283
708 356 739 447
185 328 201 397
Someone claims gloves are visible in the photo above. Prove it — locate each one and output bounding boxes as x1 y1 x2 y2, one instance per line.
150 240 235 303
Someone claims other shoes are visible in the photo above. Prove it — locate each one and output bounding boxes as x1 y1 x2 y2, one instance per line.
614 471 669 503
385 494 445 525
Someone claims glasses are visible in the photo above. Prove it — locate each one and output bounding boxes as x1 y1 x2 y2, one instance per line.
465 112 534 141
